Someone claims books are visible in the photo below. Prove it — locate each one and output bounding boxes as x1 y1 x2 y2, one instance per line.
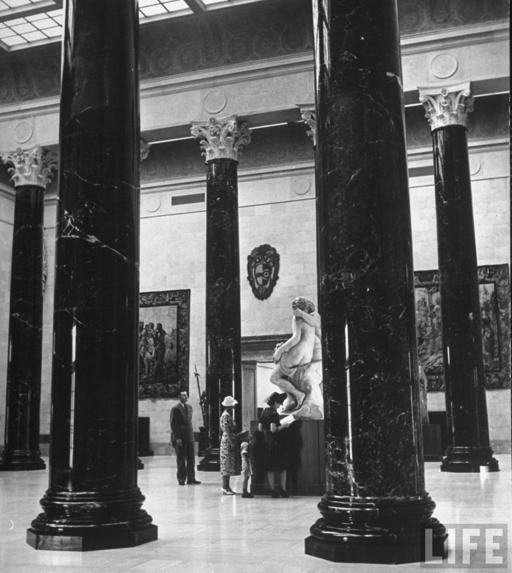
278 414 296 424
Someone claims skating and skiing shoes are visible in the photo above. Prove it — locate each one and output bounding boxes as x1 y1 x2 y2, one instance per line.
178 480 290 498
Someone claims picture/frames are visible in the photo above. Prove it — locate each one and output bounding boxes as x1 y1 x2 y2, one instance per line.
138 287 191 399
413 263 512 392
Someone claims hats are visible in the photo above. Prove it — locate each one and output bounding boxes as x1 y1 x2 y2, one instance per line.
221 395 238 406
267 391 289 406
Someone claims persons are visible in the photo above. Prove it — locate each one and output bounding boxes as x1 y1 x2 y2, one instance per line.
169 391 200 484
416 288 498 370
241 435 255 497
139 318 176 381
257 391 293 497
218 394 248 495
282 300 324 419
268 295 316 410
